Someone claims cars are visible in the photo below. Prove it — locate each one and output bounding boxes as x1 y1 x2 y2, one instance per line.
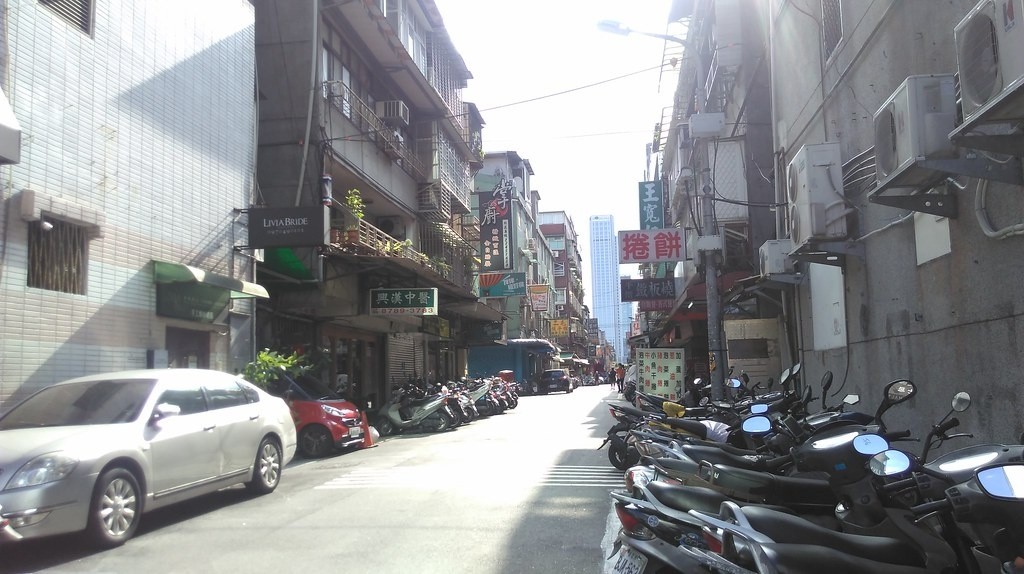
263 371 365 460
540 368 574 394
0 368 298 550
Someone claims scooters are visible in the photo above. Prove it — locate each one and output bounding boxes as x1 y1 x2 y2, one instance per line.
377 374 529 433
596 373 1024 574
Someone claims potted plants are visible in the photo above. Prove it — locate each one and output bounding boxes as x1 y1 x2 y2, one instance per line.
345 188 366 242
329 224 342 243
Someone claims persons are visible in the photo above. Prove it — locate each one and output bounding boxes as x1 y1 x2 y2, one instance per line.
594 370 599 385
610 363 625 393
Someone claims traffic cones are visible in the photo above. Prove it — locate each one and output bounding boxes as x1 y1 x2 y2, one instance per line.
357 410 380 448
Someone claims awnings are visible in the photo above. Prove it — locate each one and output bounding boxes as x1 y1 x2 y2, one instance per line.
153 261 270 298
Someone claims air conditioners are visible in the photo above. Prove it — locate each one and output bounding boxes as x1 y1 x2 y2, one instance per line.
786 142 849 254
528 238 535 251
376 100 409 127
421 186 438 209
871 73 957 196
953 0 1024 122
759 239 797 277
378 215 404 235
